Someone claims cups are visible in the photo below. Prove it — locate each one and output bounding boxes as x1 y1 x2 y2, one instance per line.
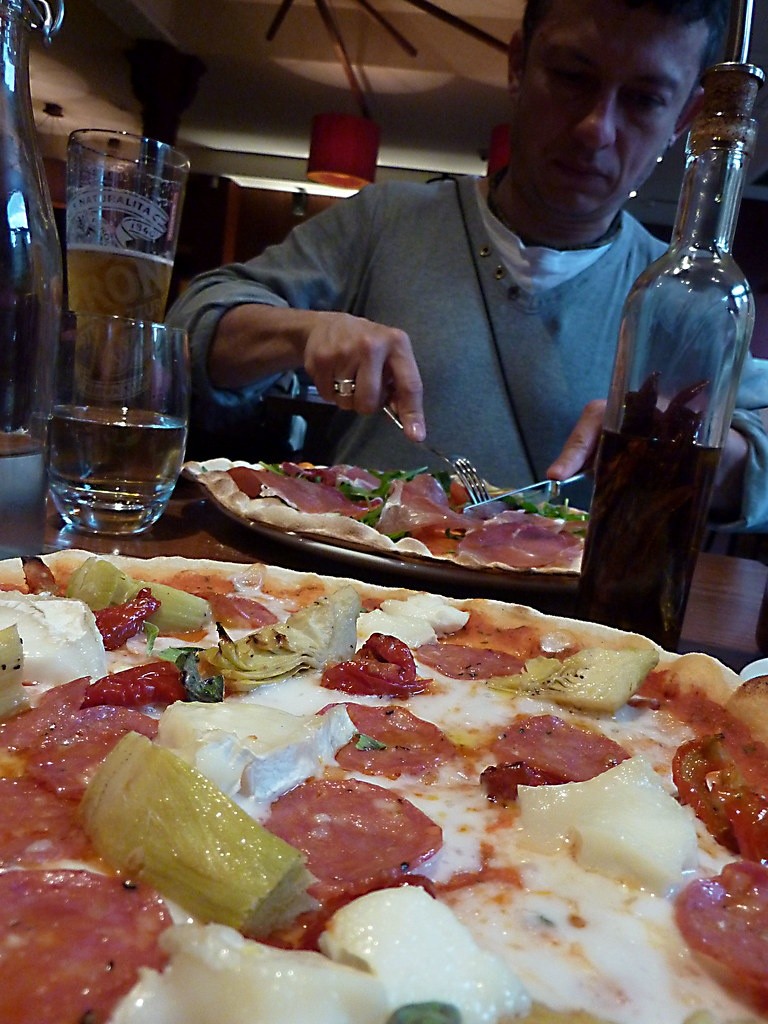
51 314 190 536
64 129 191 323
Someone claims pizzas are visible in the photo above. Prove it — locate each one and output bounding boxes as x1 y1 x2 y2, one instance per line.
181 457 591 574
0 550 768 1024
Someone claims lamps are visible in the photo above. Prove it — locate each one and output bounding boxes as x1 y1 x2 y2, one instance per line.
305 113 381 189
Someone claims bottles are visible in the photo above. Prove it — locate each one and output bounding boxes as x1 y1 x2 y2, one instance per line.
0 0 64 455
576 60 766 651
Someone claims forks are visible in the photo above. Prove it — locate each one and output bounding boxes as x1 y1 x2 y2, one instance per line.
381 402 489 503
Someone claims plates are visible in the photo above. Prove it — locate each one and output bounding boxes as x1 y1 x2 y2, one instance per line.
184 469 579 596
0 549 745 693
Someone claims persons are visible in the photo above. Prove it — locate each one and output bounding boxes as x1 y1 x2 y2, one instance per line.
163 0 768 547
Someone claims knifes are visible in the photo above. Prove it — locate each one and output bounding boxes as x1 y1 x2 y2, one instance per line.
463 470 593 517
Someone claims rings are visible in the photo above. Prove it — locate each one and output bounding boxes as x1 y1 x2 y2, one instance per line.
332 378 357 398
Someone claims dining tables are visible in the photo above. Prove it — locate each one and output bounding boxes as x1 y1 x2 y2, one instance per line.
0 422 768 1024
